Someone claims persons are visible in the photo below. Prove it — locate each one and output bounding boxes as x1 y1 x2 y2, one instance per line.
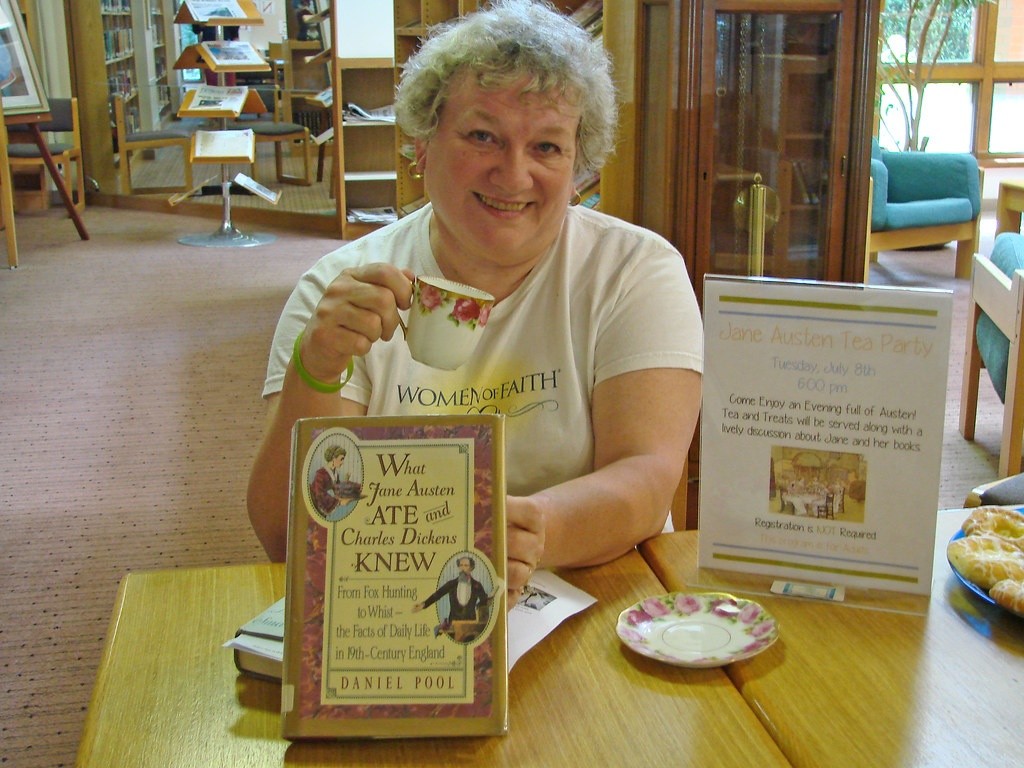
245 1 704 609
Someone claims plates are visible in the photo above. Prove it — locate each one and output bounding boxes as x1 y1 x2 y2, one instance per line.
615 591 779 668
946 504 1024 617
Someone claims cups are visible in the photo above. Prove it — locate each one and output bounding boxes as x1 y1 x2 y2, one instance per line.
394 275 496 371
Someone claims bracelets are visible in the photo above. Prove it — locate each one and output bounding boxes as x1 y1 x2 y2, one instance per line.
294 331 354 393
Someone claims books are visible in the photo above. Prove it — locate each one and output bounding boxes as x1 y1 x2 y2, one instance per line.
222 598 285 682
565 1 604 49
280 414 510 740
571 169 601 210
101 0 169 154
150 0 168 107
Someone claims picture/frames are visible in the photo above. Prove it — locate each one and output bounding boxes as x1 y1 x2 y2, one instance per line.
0 0 50 115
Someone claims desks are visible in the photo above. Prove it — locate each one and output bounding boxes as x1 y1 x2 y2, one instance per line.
994 179 1024 236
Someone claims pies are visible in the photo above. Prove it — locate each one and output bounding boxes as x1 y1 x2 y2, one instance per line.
947 505 1024 616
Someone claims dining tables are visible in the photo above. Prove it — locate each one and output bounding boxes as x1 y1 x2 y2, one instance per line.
74 505 1024 768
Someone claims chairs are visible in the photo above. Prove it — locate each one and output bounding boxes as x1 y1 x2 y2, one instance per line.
227 84 312 187
959 232 1024 478
862 137 984 280
235 61 279 121
111 91 194 198
7 97 86 218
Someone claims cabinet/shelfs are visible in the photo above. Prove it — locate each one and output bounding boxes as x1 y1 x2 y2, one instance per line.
332 55 396 241
166 0 284 247
678 0 880 315
70 0 173 196
393 0 602 217
